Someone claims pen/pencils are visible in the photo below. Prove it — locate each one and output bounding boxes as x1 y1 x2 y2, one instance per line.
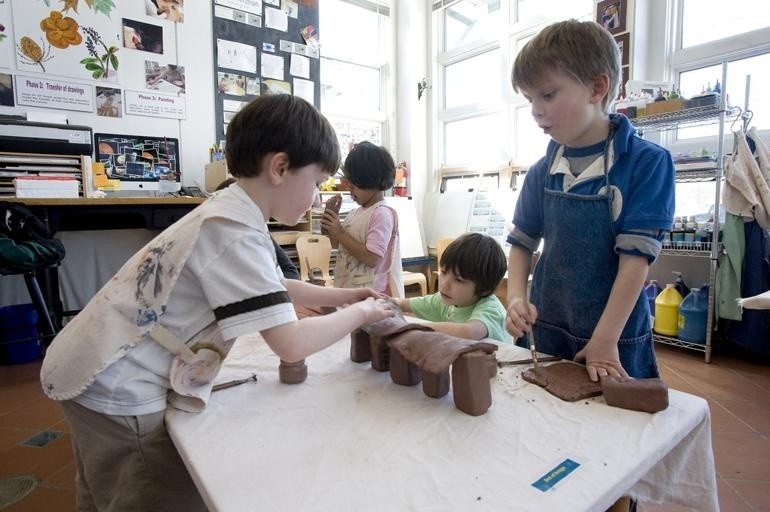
499 356 563 366
529 324 539 364
213 378 251 394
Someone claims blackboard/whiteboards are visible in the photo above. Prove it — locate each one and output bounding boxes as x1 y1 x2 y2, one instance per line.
467 181 542 251
320 192 427 258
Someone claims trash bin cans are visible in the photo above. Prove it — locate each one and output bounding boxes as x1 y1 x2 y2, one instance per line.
1 304 44 362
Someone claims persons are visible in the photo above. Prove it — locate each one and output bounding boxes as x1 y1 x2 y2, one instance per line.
218 72 260 95
145 1 171 19
504 17 677 512
133 23 162 54
36 92 397 510
390 232 516 346
319 139 408 302
213 177 302 281
144 60 186 91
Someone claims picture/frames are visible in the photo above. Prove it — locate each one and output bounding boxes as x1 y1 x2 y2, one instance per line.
94 132 180 183
593 0 635 80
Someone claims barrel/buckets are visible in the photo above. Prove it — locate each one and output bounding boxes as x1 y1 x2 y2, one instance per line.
643 270 710 345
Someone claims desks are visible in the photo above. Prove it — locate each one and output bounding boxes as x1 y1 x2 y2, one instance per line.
165 316 710 511
0 197 203 334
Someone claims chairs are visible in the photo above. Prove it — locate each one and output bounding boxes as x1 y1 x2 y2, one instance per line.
0 200 66 348
296 234 540 297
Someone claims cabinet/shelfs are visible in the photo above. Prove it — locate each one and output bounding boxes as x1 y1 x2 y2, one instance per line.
609 61 754 365
